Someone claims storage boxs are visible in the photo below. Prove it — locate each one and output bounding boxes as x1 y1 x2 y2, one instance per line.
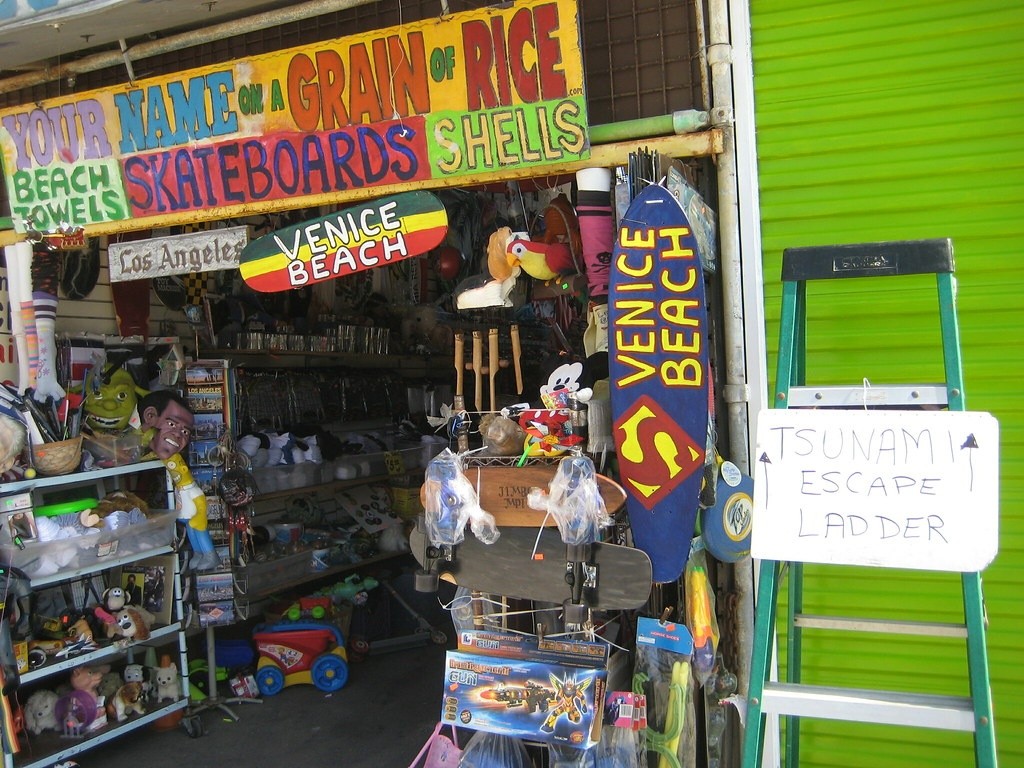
351 590 392 640
0 508 181 581
203 638 255 668
412 441 483 467
252 458 338 496
336 439 426 479
84 434 144 467
392 486 425 518
234 544 314 596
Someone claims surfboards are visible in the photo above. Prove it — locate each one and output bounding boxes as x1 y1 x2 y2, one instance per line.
606 185 712 583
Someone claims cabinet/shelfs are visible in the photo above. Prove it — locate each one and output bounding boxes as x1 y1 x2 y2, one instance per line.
1 460 203 768
196 338 583 608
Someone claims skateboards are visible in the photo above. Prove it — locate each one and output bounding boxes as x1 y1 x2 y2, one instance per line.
238 189 451 294
408 509 655 625
419 466 628 527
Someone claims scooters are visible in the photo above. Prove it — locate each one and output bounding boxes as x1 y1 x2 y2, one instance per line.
347 565 450 654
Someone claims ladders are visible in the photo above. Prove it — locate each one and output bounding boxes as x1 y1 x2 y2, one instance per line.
740 236 1004 768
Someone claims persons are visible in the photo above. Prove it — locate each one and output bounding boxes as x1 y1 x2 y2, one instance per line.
137 390 195 461
122 574 142 607
143 570 164 608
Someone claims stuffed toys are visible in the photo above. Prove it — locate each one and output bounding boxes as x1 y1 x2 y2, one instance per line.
456 225 609 458
23 491 180 730
